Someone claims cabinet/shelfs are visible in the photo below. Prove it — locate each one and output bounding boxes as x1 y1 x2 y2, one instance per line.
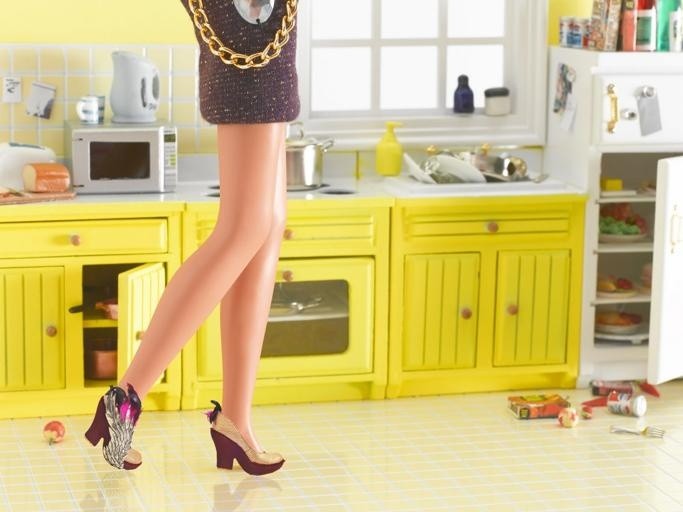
0 203 182 421
546 46 683 386
385 195 587 399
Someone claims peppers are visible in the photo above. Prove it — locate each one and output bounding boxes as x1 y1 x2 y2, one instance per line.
599 275 632 292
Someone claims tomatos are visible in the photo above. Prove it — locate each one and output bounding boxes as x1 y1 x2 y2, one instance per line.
43 421 66 446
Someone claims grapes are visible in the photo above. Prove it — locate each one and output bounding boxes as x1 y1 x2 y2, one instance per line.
600 204 647 235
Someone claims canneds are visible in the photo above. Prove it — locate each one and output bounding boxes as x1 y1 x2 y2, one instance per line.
607 391 648 417
559 17 590 49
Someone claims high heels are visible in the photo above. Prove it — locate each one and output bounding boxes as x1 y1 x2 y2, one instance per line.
84 393 143 472
209 409 287 478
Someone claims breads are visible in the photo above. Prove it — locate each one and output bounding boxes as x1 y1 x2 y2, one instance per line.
22 162 70 193
